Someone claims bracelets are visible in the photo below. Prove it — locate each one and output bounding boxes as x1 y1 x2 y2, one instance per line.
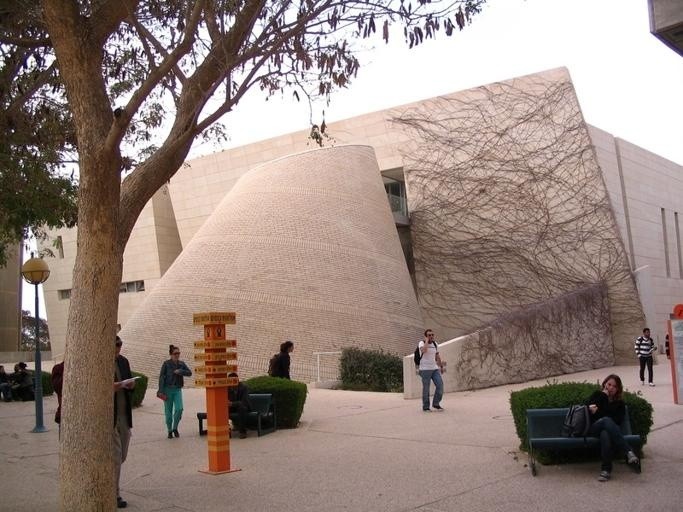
441 366 443 368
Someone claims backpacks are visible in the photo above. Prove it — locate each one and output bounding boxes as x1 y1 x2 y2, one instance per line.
414 341 437 365
560 404 591 448
267 355 282 376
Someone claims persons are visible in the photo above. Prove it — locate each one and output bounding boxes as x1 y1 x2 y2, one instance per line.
159 345 192 438
114 336 136 507
419 329 444 412
227 373 252 438
587 375 639 482
0 362 33 401
277 342 294 380
635 328 655 386
52 362 64 442
665 334 670 359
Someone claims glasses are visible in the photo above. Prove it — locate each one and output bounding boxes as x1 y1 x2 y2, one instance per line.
171 351 180 355
116 342 122 347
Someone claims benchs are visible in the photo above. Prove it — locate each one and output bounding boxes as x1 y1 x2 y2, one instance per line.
523 402 645 481
194 393 277 438
0 375 38 403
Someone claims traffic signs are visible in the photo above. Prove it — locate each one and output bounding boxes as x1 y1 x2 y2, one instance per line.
189 307 243 389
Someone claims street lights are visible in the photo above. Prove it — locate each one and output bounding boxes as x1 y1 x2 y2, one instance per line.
18 255 52 434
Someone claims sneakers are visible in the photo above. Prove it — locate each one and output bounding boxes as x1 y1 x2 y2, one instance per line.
239 430 246 439
117 497 127 508
640 381 655 386
626 450 640 465
168 430 179 438
423 404 443 412
597 470 611 482
